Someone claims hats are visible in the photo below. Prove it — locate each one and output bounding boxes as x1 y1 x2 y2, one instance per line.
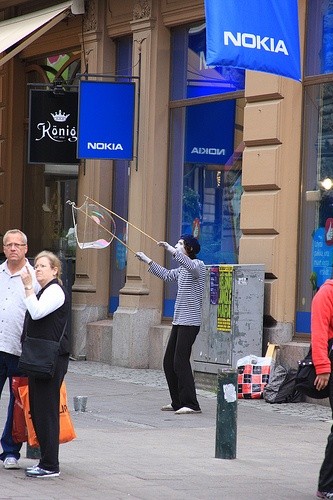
181 234 201 254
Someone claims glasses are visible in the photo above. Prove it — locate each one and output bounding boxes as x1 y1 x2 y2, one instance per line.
4 243 25 248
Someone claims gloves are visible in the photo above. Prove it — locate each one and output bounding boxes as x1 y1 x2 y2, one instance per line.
134 252 153 264
157 241 177 255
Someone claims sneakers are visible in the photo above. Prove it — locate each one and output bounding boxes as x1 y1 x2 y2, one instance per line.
2 457 19 470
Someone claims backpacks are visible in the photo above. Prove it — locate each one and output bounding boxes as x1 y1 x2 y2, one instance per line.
262 364 302 403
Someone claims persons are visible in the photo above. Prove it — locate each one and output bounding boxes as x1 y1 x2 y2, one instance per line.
19 250 71 478
134 234 206 414
0 229 42 469
310 277 333 500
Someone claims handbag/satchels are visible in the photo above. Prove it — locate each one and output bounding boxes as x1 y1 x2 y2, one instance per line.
236 355 272 399
17 380 76 448
12 377 29 443
294 340 330 400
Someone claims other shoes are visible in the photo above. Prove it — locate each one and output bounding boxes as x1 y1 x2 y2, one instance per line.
161 404 176 411
25 467 61 478
174 406 202 414
25 463 40 471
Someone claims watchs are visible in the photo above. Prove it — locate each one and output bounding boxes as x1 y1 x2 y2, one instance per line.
24 285 33 290
